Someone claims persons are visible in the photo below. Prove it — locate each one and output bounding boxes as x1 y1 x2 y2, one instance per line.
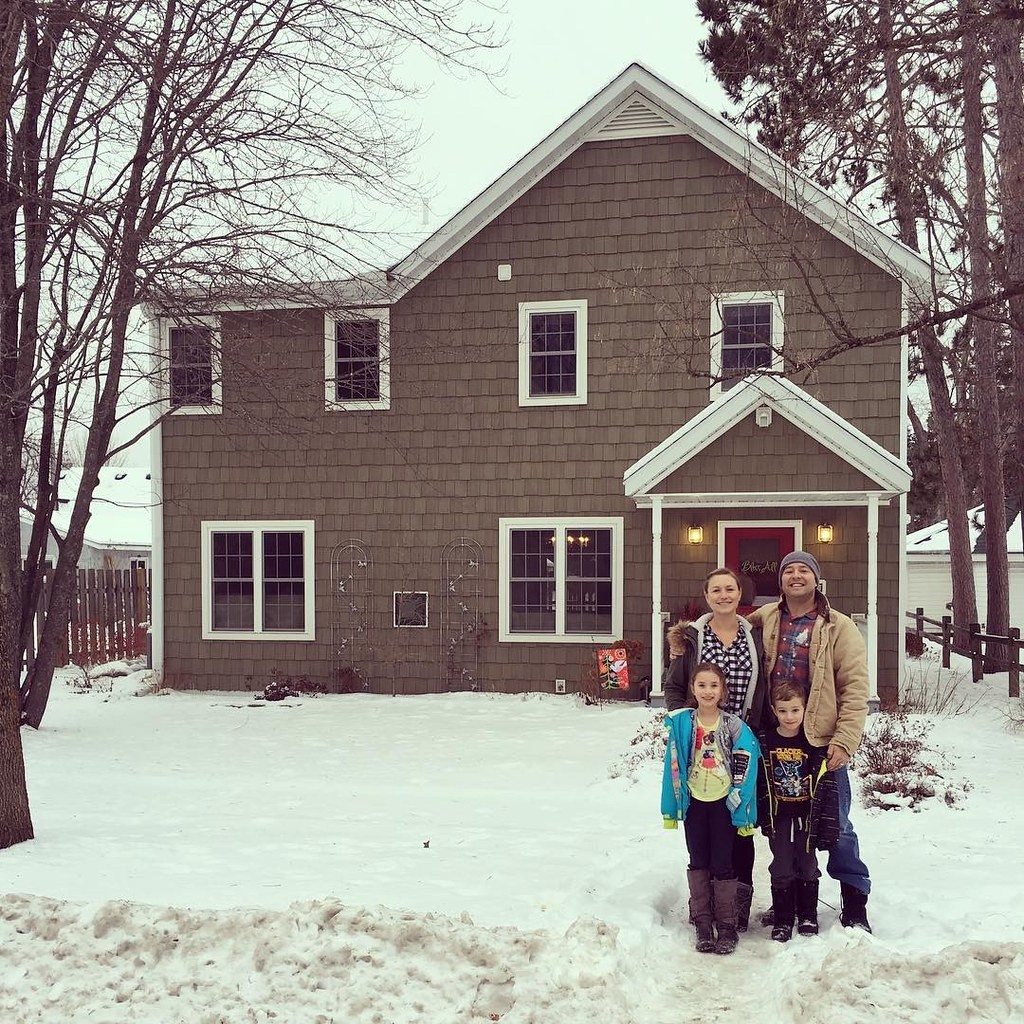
661 662 760 954
663 567 764 933
754 679 840 942
670 551 873 937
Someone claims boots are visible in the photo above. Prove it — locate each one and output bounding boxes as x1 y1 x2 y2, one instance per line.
688 869 715 953
762 905 775 926
772 886 798 942
713 877 739 954
797 882 818 935
841 884 871 935
739 882 753 932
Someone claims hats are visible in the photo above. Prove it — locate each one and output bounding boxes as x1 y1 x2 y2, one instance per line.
779 552 820 589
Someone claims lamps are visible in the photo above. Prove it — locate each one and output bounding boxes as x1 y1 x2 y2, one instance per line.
818 522 833 543
687 523 703 544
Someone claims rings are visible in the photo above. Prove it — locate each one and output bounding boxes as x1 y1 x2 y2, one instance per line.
840 763 844 766
